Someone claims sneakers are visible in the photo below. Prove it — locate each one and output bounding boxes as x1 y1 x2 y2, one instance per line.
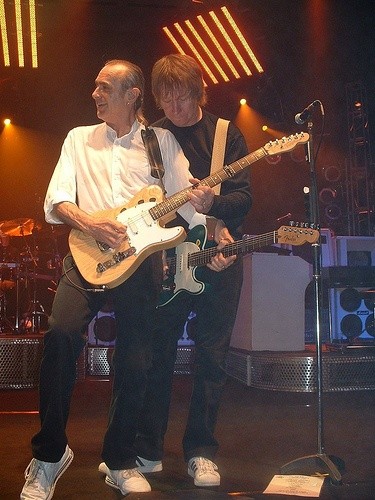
19 444 74 500
98 457 163 474
187 456 220 486
104 469 151 496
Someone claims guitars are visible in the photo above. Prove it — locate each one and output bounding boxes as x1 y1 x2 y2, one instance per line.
68 131 310 292
154 220 321 310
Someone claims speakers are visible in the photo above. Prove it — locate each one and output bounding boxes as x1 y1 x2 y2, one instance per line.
176 312 199 347
284 229 375 341
85 311 118 347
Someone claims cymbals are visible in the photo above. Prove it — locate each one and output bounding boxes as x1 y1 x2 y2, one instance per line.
0 217 43 236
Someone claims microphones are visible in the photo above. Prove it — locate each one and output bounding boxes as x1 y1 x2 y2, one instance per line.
295 100 319 124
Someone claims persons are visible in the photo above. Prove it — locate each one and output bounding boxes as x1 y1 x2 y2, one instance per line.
19 60 237 500
98 54 253 487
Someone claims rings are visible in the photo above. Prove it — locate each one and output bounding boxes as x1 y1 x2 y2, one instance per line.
201 200 207 205
201 205 206 211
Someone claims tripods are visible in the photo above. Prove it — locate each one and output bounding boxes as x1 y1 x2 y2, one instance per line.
0 234 62 334
275 121 345 486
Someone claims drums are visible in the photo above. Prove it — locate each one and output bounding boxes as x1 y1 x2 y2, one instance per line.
21 252 63 279
